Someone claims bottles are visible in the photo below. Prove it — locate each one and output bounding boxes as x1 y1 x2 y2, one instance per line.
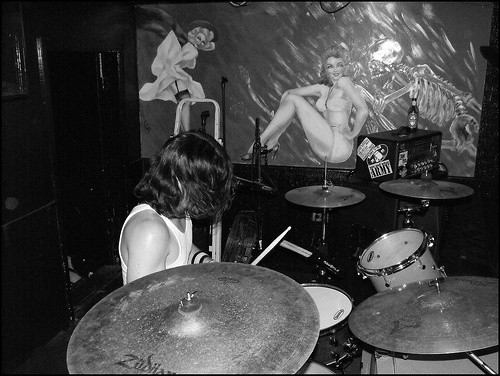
407 93 419 133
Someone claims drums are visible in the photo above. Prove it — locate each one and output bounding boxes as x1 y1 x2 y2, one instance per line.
300 283 355 372
356 229 447 292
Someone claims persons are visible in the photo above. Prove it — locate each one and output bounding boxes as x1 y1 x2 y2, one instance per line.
118 129 233 286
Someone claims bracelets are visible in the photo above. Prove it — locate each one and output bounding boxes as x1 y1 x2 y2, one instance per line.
191 251 209 264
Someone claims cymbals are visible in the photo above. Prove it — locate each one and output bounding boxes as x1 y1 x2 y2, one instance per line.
379 179 475 200
67 262 320 374
285 186 365 208
349 275 498 354
